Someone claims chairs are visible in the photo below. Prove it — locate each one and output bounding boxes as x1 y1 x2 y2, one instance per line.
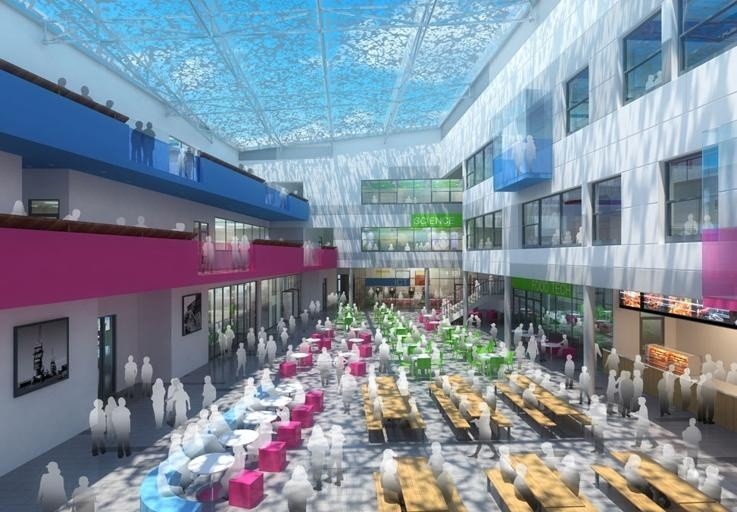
337 305 514 378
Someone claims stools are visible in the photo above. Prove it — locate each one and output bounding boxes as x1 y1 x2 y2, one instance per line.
228 328 372 507
564 347 576 359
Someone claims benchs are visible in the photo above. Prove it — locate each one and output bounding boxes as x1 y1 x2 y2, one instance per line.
138 409 235 512
360 372 668 512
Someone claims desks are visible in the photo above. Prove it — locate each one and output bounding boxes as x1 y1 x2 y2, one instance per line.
186 428 259 503
609 449 733 511
512 329 562 363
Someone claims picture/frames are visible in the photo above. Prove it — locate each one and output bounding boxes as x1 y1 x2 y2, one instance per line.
182 292 202 336
12 316 70 397
